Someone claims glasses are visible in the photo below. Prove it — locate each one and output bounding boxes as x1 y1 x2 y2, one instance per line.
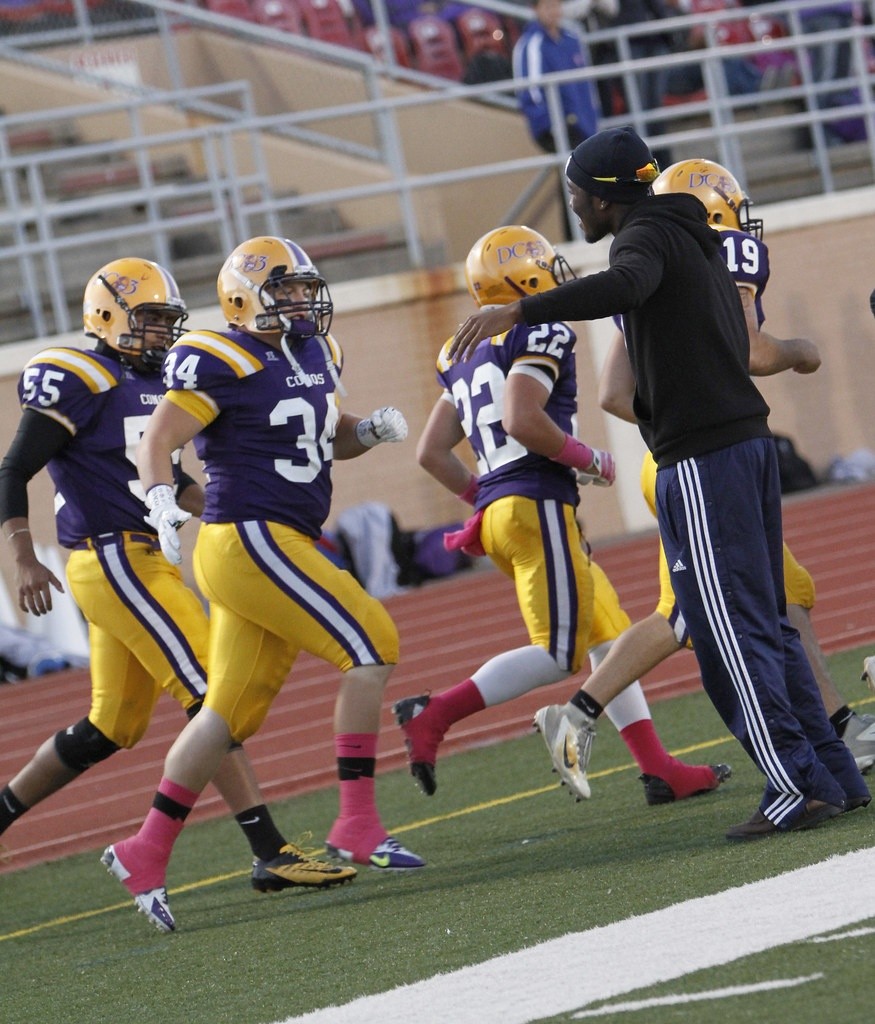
637 158 659 184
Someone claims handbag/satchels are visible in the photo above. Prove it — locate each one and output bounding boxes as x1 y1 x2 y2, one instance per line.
773 433 818 495
391 521 472 588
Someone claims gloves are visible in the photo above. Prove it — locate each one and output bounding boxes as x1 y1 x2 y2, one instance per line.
588 445 616 486
144 483 192 566
355 406 409 450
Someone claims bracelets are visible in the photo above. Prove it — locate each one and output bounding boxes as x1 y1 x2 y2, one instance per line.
3 527 30 541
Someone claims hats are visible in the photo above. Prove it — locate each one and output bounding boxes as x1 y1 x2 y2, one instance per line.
565 126 660 205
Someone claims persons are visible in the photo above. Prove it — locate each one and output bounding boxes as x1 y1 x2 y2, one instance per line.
444 123 871 839
454 0 874 241
1 258 357 893
389 224 732 806
98 236 426 933
531 158 875 801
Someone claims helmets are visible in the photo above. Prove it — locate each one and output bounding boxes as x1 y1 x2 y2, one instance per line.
217 236 334 340
466 224 578 310
652 158 764 242
81 256 192 371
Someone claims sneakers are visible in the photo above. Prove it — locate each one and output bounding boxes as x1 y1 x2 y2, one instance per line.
639 753 732 805
99 834 175 936
533 703 598 801
250 841 359 893
390 689 440 798
327 814 427 869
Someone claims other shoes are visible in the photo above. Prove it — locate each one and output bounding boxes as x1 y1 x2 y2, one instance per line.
838 713 875 774
725 796 871 840
860 654 875 694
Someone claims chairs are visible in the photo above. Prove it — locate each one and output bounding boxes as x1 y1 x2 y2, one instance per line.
0 0 875 114
